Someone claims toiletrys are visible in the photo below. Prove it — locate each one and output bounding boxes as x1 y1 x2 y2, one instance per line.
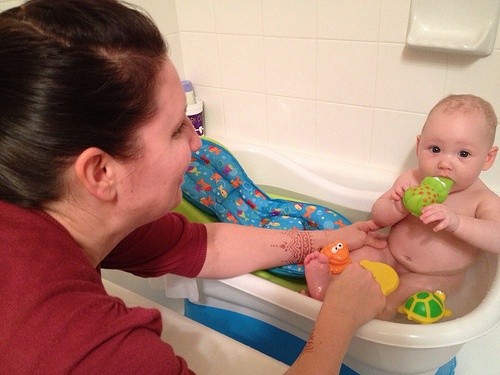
181 80 195 104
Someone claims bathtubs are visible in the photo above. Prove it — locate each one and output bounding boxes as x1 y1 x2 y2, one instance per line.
140 141 499 374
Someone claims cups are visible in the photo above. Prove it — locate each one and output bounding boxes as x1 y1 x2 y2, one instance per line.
185 97 204 136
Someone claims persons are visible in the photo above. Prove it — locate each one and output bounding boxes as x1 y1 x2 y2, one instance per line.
0 0 389 375
303 94 500 321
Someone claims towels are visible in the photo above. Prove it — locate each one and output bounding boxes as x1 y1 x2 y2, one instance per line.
128 273 199 303
